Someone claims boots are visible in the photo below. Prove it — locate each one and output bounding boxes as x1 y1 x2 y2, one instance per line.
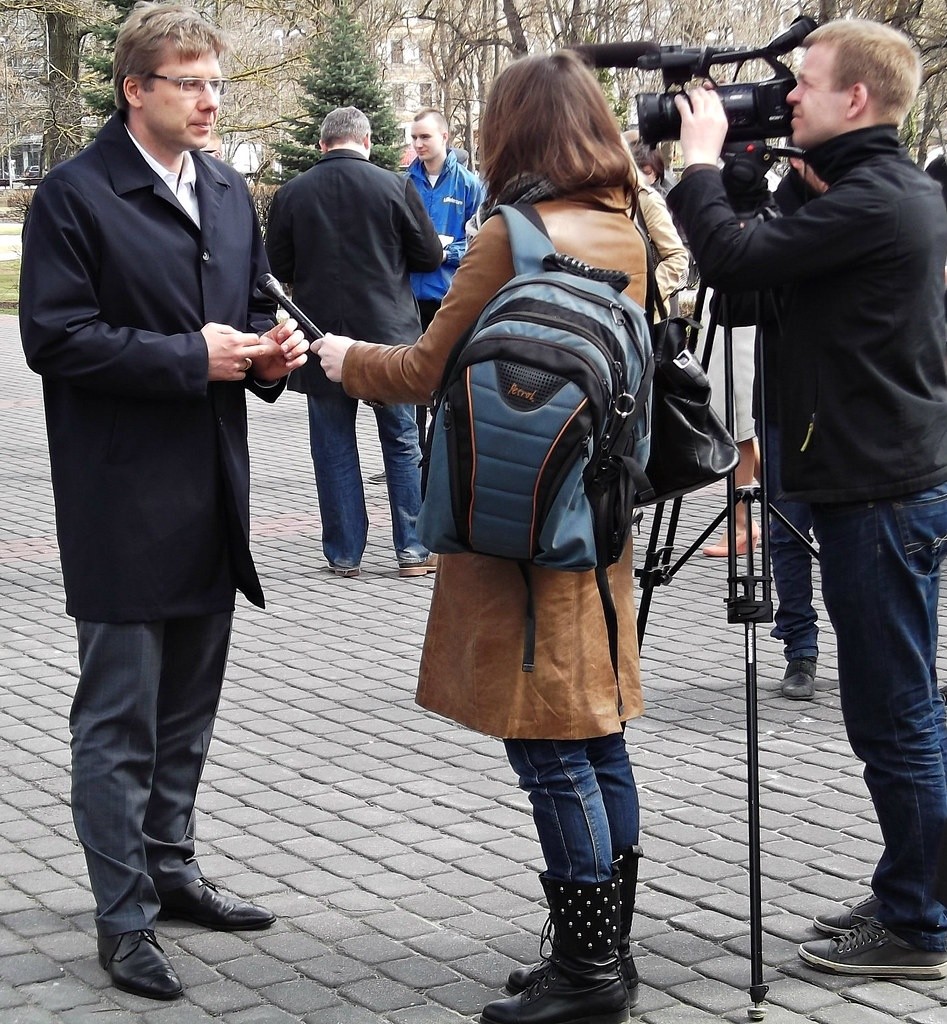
479 865 630 1024
505 845 644 1009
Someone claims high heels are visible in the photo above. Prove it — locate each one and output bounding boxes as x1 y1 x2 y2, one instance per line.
703 519 759 556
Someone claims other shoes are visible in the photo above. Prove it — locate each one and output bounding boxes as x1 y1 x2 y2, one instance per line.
366 470 388 484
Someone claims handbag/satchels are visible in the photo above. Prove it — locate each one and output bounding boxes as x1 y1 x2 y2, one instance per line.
634 222 741 508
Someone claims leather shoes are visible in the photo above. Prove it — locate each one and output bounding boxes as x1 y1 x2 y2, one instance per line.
328 561 360 576
399 552 440 576
97 930 184 997
156 877 276 932
780 656 816 699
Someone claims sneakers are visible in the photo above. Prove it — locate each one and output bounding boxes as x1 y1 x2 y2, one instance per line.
813 894 885 934
797 917 947 979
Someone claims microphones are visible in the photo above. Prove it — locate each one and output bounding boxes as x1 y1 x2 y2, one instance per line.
561 40 659 67
256 272 326 341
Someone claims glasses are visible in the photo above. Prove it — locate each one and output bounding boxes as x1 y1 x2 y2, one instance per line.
149 73 231 97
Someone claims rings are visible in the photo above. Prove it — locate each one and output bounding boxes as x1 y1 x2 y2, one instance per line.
244 360 250 372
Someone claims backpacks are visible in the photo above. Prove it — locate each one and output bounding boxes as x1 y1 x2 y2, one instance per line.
414 202 656 572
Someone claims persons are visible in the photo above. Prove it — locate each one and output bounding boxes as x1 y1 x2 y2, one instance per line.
623 131 700 325
311 53 644 1024
19 5 310 999
265 106 443 577
367 109 485 484
661 19 947 980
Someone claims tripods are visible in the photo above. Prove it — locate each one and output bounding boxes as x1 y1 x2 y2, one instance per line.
634 138 817 1020
